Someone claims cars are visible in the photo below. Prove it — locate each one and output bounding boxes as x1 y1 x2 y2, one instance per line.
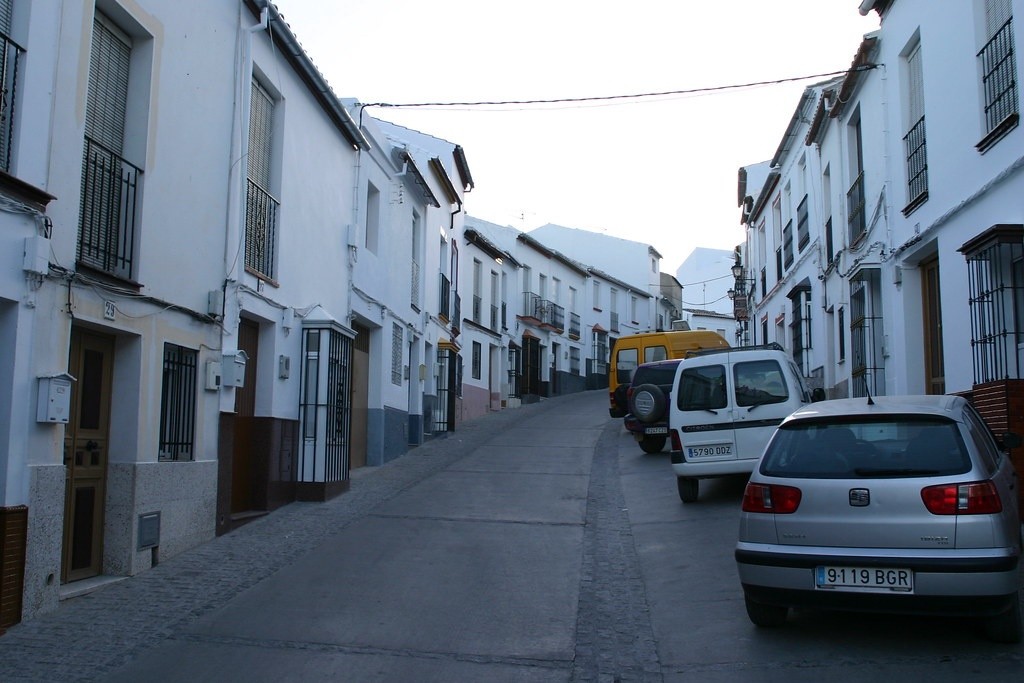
732 394 1024 644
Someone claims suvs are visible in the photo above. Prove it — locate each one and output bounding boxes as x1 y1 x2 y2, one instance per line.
665 342 827 503
623 357 686 454
606 330 731 418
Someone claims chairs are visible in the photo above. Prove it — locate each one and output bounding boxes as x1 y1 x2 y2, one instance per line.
800 428 862 472
907 427 967 471
